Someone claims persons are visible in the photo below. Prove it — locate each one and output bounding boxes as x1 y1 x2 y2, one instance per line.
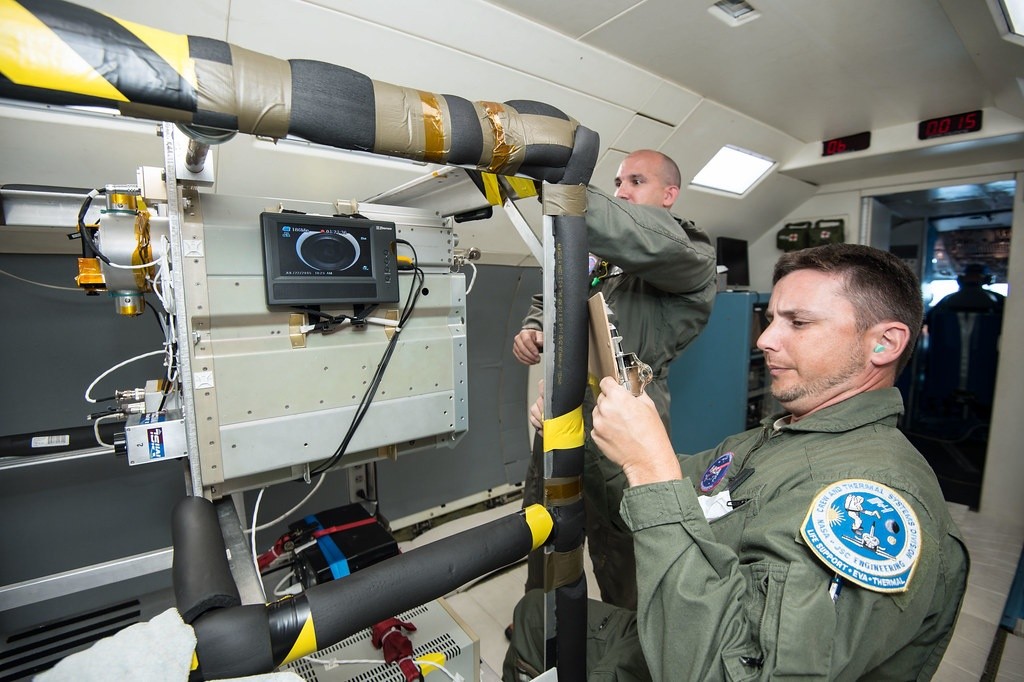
502 245 971 682
922 264 1006 337
504 151 716 642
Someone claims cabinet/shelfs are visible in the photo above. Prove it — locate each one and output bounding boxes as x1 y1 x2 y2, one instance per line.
664 291 779 453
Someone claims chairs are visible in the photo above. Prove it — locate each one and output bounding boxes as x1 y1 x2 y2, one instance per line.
924 307 996 423
